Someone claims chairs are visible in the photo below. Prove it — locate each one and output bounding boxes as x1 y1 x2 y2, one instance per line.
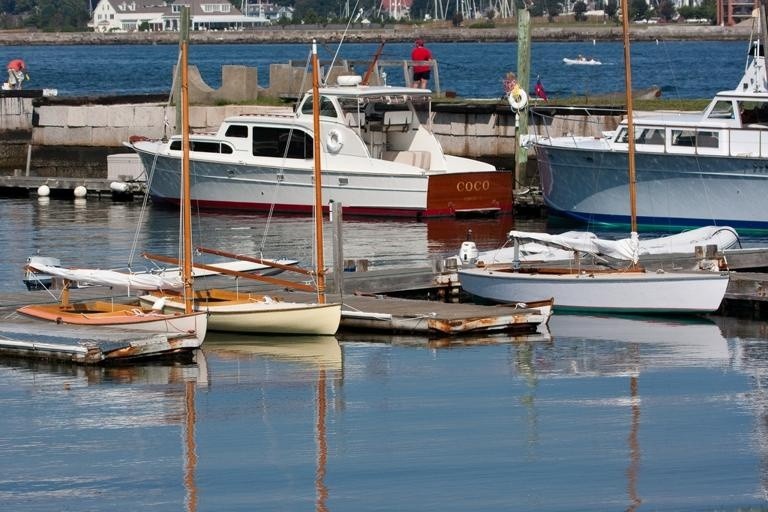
345 112 366 136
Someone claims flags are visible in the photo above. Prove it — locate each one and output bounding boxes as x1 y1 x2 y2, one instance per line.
536 79 547 101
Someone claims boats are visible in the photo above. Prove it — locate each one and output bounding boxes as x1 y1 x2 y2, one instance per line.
138 39 343 339
21 252 299 291
457 0 732 317
444 230 599 264
122 73 515 224
522 0 766 243
16 37 208 350
519 223 739 261
563 57 601 65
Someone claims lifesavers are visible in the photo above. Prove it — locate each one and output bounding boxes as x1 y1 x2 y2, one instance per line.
508 88 528 111
326 128 343 154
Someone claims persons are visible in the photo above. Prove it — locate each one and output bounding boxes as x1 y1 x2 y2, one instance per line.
576 53 593 62
7 56 25 90
502 71 517 101
411 40 432 89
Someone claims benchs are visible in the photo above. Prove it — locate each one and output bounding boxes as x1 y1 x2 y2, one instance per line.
370 111 412 132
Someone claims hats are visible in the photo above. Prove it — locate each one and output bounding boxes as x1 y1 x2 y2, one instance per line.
416 40 424 45
